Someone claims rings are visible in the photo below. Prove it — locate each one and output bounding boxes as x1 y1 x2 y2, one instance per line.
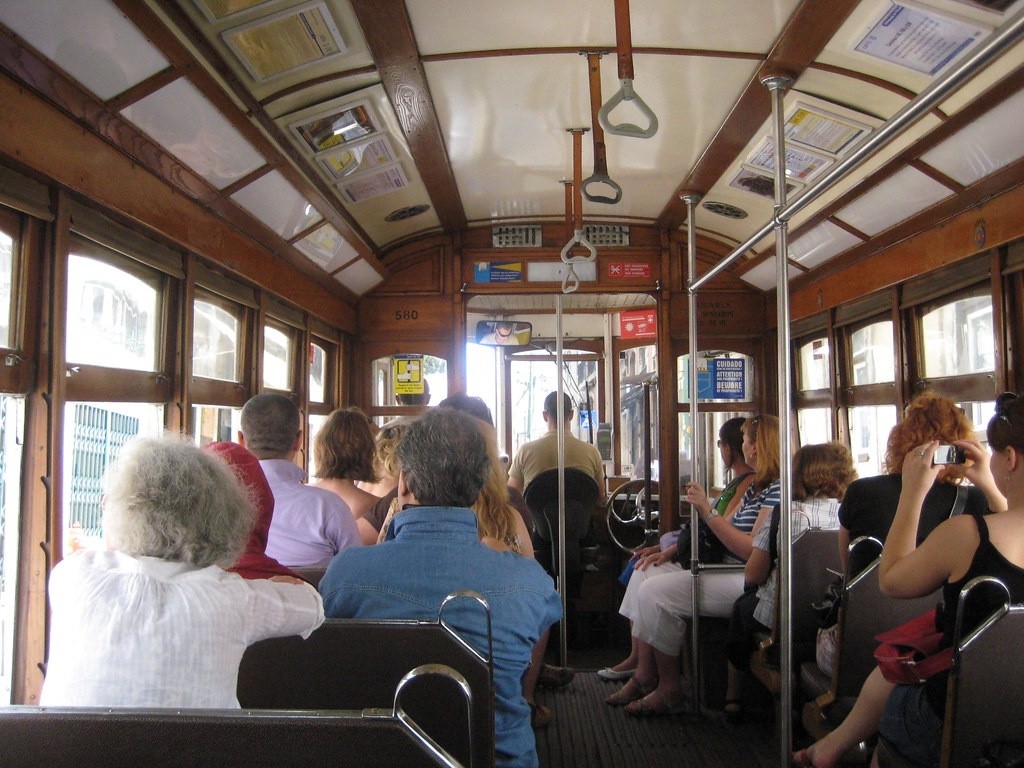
914 452 924 456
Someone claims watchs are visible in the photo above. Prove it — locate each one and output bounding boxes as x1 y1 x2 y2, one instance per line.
703 509 719 524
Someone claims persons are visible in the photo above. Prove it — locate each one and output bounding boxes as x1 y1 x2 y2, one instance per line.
791 385 1024 768
205 441 305 583
237 394 365 567
318 405 563 768
354 393 554 730
380 378 432 426
305 406 386 522
359 422 411 498
507 391 606 570
479 321 519 345
723 440 859 715
596 417 757 683
607 415 797 715
377 413 535 558
40 426 326 709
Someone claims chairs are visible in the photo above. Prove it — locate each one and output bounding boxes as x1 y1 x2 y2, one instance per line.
522 467 601 640
683 527 1024 768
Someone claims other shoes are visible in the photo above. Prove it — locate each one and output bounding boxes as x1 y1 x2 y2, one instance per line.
724 697 749 720
597 666 638 679
531 702 550 727
790 749 811 768
539 663 572 687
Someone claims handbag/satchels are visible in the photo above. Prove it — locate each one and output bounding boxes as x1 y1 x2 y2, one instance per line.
873 607 950 685
659 529 682 551
676 517 723 570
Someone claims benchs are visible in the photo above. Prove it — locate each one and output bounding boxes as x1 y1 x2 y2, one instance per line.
0 563 495 767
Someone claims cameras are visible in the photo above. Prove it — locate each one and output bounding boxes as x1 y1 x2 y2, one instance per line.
932 445 966 465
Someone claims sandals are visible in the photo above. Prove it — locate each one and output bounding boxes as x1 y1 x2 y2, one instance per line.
623 698 685 715
606 675 659 704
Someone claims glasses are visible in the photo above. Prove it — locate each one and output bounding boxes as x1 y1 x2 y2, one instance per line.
752 417 760 441
994 392 1014 445
717 440 727 447
